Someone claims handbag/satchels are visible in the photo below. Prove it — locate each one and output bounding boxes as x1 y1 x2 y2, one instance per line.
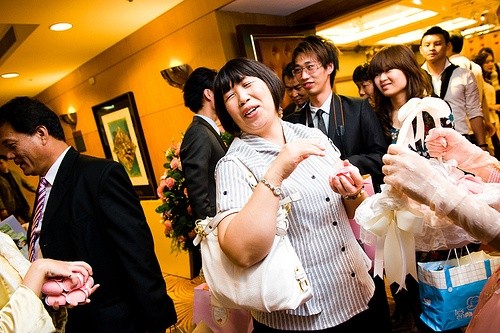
195 193 318 311
416 246 500 332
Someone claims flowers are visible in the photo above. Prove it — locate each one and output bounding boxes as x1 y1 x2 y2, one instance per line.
155 117 234 254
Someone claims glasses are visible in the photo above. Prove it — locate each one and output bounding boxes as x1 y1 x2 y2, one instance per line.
292 63 323 76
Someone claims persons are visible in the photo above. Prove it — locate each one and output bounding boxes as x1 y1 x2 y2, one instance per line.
282 62 310 123
0 157 36 224
0 96 177 333
353 45 452 333
419 26 500 162
214 57 376 333
179 67 227 219
382 128 500 333
0 231 99 333
292 35 391 333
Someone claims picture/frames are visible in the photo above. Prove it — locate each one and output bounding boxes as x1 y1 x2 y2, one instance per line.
92 91 158 199
236 24 317 112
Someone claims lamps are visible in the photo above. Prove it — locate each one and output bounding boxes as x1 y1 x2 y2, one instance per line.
162 65 189 88
60 112 77 126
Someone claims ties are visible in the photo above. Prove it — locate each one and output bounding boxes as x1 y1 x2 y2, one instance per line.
27 178 48 262
315 109 328 138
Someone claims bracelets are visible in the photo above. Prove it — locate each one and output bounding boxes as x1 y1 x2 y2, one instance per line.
343 186 365 201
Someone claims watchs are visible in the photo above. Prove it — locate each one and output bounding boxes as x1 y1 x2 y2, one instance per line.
261 179 283 195
